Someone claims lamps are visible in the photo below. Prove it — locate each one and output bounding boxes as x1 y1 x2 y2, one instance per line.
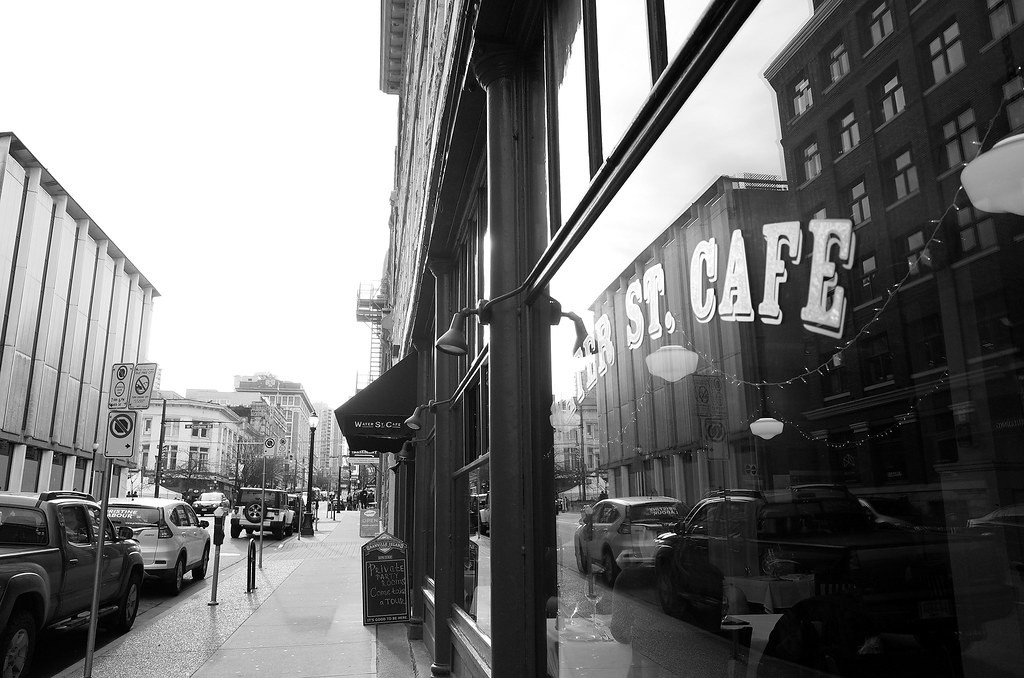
961 133 1024 216
750 418 784 440
388 459 402 475
403 400 436 429
398 438 417 458
436 299 491 356
549 297 600 357
646 345 699 382
550 411 581 433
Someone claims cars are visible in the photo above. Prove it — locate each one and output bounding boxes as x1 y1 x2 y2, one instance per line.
94 492 217 595
192 491 230 516
288 494 306 532
319 491 329 502
652 482 922 640
967 506 1024 566
573 496 692 586
328 499 346 511
477 490 490 535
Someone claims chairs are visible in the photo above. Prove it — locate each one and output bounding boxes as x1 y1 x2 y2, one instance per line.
3 516 37 542
816 571 857 594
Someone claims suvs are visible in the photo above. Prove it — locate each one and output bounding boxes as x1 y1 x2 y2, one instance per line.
0 487 145 678
230 486 295 540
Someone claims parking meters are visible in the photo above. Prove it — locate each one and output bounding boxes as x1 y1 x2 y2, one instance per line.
207 507 228 606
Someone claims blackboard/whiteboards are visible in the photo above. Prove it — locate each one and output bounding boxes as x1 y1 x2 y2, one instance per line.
361 531 411 626
463 539 479 623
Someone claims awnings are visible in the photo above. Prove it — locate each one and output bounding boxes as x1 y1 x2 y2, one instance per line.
333 350 417 453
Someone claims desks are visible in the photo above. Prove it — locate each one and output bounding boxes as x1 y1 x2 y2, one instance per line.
727 573 856 614
547 614 822 677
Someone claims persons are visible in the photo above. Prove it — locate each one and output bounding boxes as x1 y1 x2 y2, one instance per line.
609 508 726 678
353 492 359 511
126 491 141 497
359 487 368 509
346 493 352 507
368 490 375 508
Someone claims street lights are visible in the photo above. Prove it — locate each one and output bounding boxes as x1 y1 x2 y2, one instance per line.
299 409 319 537
87 441 100 495
